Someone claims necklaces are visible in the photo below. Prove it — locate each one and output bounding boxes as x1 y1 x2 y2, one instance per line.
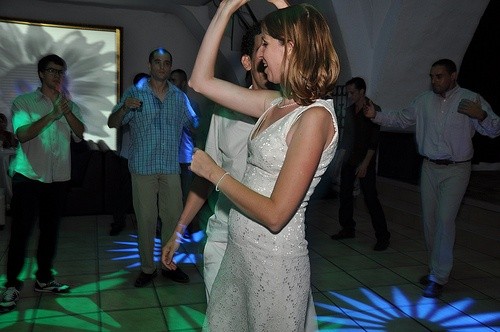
278 101 297 108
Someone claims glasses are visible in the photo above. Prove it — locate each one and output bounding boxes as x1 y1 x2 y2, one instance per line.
45 67 66 77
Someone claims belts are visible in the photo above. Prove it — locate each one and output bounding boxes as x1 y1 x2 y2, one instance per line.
424 156 470 166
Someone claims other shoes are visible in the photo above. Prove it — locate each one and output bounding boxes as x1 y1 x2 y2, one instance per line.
374 238 390 251
420 275 428 283
110 222 125 236
161 267 189 282
423 281 441 298
331 232 355 240
135 270 156 288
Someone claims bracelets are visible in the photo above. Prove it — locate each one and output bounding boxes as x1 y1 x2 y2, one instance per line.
216 173 230 191
482 111 487 119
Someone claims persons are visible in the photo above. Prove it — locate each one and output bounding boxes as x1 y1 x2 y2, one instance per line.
169 68 203 234
188 0 339 332
331 77 390 251
0 113 18 148
162 20 319 332
363 58 500 300
0 54 86 314
108 48 210 287
109 72 151 236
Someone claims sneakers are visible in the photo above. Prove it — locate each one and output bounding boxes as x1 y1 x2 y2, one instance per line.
0 287 23 313
33 279 69 294
